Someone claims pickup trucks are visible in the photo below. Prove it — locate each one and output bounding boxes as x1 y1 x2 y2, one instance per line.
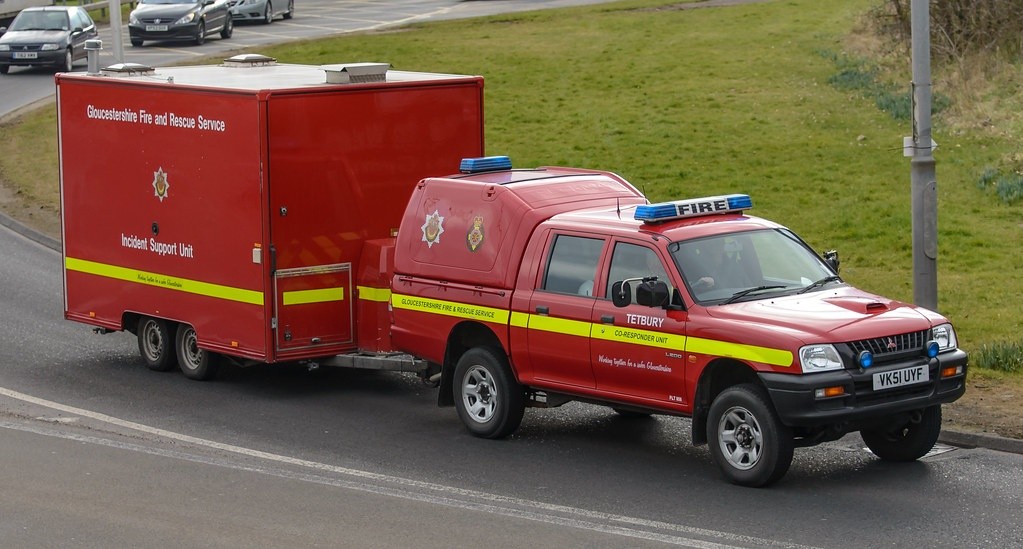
387 157 968 490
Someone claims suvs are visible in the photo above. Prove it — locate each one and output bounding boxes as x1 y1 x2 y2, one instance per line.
128 0 234 48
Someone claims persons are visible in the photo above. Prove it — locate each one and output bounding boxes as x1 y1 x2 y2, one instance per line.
691 240 753 291
24 12 48 28
609 253 711 305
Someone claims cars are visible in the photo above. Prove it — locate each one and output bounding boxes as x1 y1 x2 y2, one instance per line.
0 5 99 76
229 0 295 24
